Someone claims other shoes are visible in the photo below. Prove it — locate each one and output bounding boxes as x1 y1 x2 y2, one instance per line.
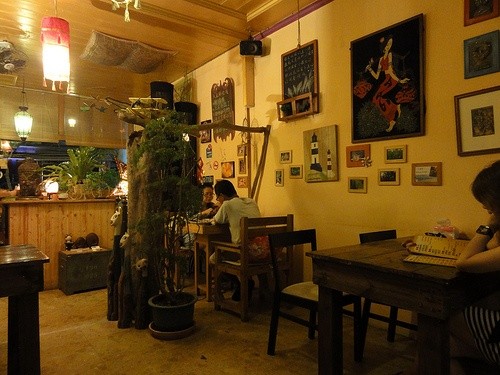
232 279 255 302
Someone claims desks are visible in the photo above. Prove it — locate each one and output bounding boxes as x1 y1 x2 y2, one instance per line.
305 235 464 375
0 243 50 375
188 222 231 302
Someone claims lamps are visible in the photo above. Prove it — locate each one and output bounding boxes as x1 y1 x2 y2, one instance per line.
41 0 71 92
14 91 33 142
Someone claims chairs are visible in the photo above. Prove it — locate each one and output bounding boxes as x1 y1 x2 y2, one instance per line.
211 214 293 323
268 230 416 363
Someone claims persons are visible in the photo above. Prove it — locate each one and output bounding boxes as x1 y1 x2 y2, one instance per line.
402 161 500 375
189 179 267 302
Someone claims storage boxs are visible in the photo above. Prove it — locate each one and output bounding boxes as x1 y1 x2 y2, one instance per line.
58 247 113 295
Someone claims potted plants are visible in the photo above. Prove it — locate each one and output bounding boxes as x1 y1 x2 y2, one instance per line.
132 113 206 332
33 146 122 200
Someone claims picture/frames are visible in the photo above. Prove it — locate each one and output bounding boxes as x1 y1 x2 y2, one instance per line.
290 164 303 179
378 144 408 185
348 176 367 193
349 13 426 143
464 0 500 26
279 150 292 163
237 144 248 188
454 85 500 157
346 145 372 168
412 161 442 186
275 169 284 187
463 29 500 79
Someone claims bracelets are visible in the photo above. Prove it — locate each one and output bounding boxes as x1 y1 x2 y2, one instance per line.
475 225 496 237
